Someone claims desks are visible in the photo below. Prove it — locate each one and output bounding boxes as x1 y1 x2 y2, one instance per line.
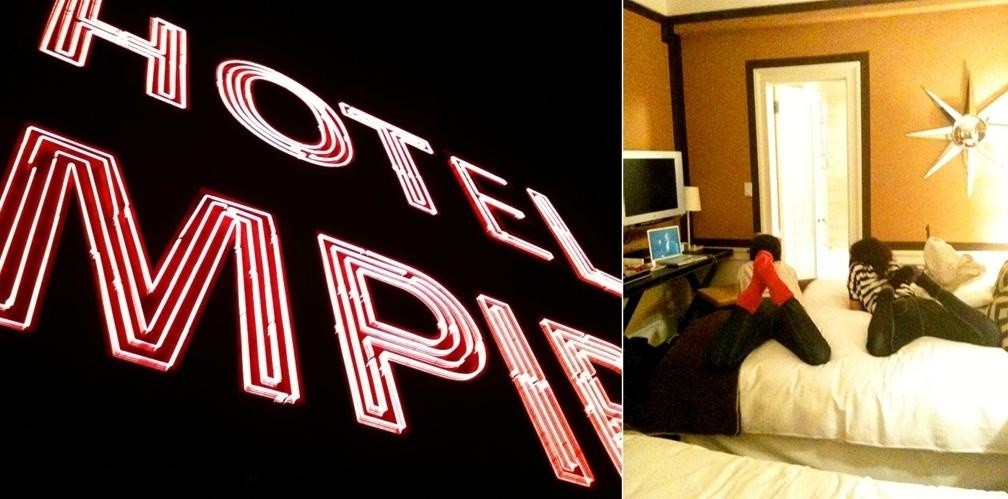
623 246 734 366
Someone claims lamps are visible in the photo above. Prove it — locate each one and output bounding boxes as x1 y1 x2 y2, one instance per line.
683 185 705 254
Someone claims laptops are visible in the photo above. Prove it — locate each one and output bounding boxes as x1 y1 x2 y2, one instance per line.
647 225 708 267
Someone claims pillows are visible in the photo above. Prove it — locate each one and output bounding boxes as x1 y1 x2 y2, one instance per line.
953 262 1004 306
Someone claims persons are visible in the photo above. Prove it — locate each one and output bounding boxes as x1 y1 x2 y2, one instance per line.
846 236 1000 359
657 232 676 258
710 229 833 379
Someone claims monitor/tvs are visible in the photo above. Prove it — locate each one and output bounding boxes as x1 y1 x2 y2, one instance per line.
622 151 686 227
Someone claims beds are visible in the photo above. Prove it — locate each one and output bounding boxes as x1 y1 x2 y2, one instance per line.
624 275 1007 498
